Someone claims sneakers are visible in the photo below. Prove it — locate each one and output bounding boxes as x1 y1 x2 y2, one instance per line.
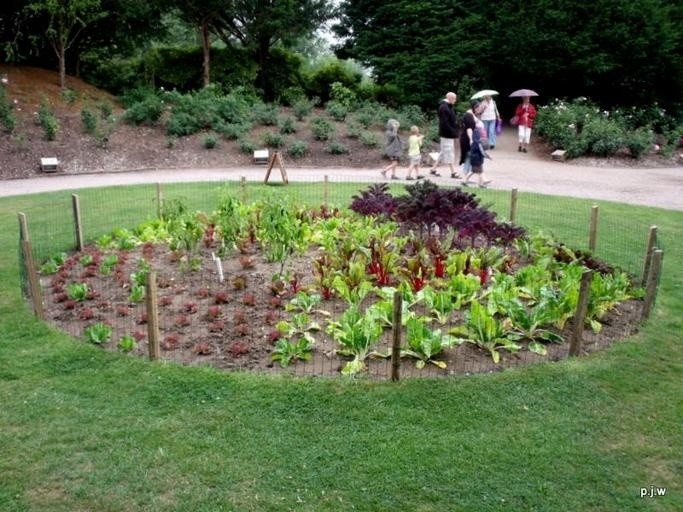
380 170 424 180
462 178 492 188
518 146 527 153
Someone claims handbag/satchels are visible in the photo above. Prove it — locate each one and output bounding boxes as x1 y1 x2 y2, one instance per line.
496 119 502 134
510 116 519 125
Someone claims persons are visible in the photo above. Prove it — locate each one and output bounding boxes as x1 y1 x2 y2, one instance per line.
479 95 501 150
514 97 537 154
457 112 493 184
429 91 460 179
467 100 488 149
379 118 403 180
457 129 490 188
405 126 426 181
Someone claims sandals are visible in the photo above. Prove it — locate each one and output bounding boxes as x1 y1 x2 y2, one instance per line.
429 168 440 176
452 173 461 178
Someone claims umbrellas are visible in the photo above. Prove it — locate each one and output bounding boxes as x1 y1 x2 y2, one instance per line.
507 89 539 98
470 89 500 100
